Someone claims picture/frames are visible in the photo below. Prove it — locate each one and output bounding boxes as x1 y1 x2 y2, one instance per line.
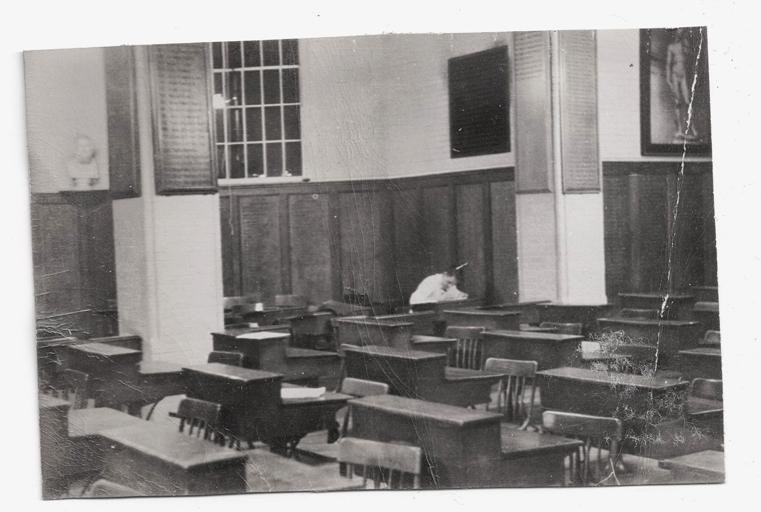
639 28 710 157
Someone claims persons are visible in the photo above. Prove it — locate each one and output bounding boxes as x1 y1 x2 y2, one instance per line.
665 30 700 142
406 264 470 304
